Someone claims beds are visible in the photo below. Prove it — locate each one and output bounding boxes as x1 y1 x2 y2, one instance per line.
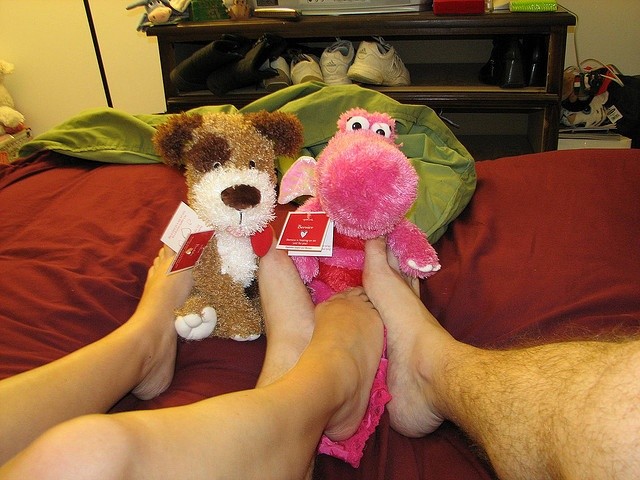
1 81 639 479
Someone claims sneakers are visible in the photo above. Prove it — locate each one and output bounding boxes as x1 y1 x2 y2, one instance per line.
320 37 375 87
347 36 412 87
290 54 325 85
258 54 293 92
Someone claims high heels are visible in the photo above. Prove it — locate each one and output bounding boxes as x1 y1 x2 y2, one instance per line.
479 40 527 88
523 36 547 86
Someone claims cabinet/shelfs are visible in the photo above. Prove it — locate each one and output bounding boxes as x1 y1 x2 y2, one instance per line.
136 7 575 161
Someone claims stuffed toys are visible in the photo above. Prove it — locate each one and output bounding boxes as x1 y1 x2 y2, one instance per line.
1 59 25 137
126 0 189 33
150 106 305 342
560 91 616 129
280 105 443 471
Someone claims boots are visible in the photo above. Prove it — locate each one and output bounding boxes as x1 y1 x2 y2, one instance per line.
206 34 287 98
163 34 254 98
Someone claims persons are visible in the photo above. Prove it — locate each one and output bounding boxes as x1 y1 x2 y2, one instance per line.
252 234 640 480
0 242 385 480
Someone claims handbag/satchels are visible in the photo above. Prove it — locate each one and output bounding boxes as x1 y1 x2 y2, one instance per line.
560 59 614 128
